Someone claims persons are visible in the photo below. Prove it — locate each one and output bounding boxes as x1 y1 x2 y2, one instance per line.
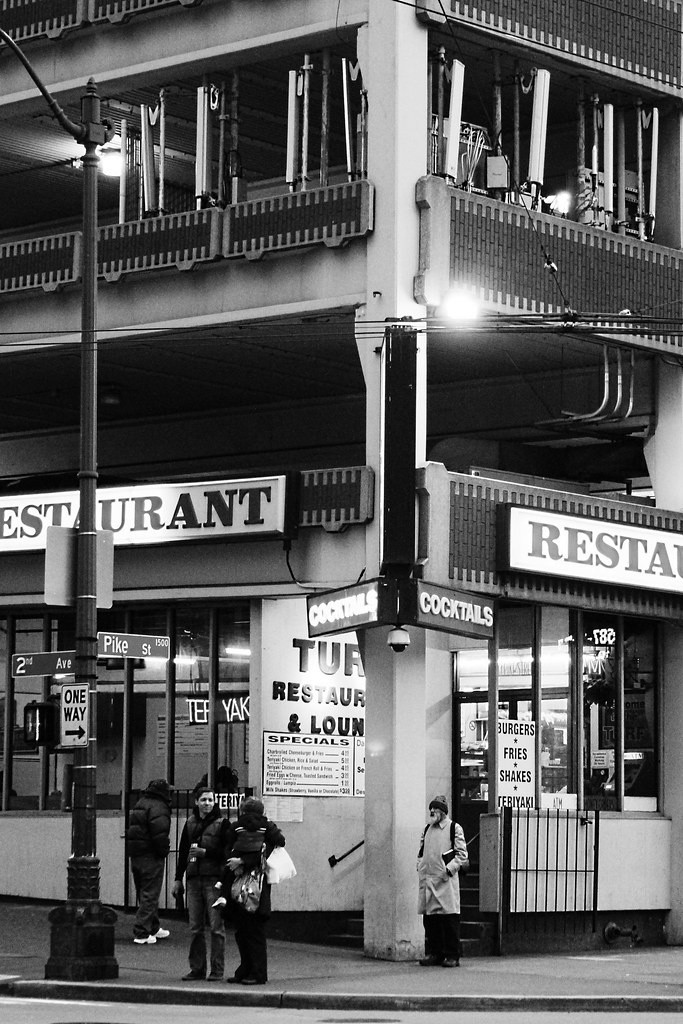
127 778 170 944
170 787 231 981
212 796 286 986
415 795 468 968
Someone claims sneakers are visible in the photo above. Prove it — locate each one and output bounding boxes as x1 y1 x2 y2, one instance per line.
133 934 157 944
206 972 223 981
152 927 170 938
181 970 206 980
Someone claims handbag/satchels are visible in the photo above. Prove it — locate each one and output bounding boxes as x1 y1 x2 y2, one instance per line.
230 842 267 914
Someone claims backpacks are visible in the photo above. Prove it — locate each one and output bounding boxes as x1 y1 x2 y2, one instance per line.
418 821 472 873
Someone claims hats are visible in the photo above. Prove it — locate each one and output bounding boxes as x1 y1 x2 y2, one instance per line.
144 778 176 793
428 794 449 815
241 799 265 816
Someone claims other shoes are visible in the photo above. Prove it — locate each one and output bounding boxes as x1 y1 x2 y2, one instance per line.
441 959 460 967
418 954 445 966
226 976 266 985
